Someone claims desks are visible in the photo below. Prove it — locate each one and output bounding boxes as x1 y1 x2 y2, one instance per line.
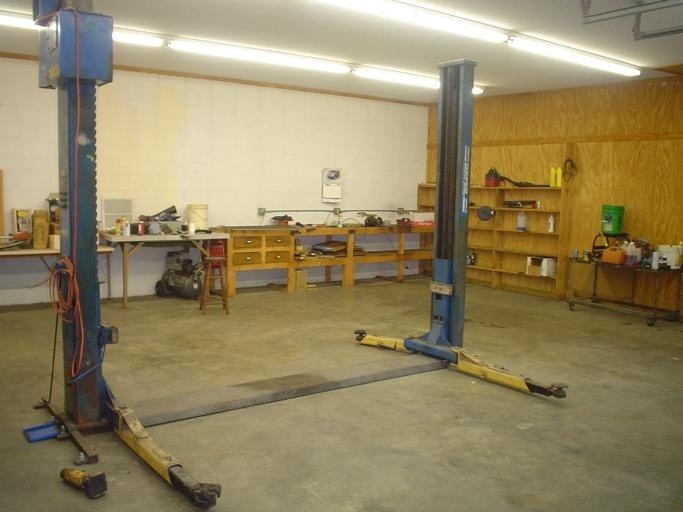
100 233 230 310
0 245 114 298
566 255 683 327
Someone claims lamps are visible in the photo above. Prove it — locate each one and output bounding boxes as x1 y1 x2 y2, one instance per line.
1 11 166 50
166 37 349 74
507 32 642 77
350 63 484 94
327 0 508 42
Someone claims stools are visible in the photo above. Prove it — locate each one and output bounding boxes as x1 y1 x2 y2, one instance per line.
199 256 231 314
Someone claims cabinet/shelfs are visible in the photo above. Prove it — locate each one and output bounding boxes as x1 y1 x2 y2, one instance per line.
418 180 570 300
214 222 434 297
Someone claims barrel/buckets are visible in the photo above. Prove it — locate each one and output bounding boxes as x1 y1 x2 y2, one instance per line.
550 166 562 188
187 205 209 231
622 241 637 266
608 248 625 264
517 212 528 230
600 204 623 233
660 245 679 265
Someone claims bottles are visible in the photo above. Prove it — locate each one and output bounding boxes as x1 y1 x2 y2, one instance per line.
572 248 578 258
550 167 563 187
137 220 145 236
651 251 660 270
527 256 542 276
115 216 131 237
338 214 342 228
